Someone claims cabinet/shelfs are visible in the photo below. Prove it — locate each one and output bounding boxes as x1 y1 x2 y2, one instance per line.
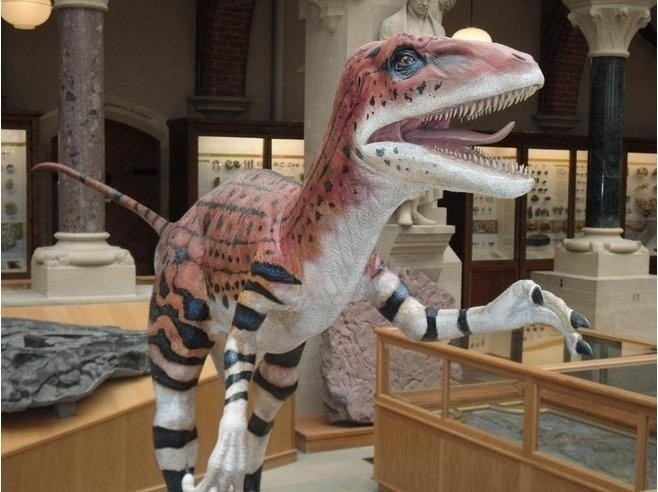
447 130 572 308
567 135 587 237
170 119 305 221
373 325 657 492
1 108 45 279
621 137 657 274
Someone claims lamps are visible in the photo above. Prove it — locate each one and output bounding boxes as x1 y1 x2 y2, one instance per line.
1 0 52 30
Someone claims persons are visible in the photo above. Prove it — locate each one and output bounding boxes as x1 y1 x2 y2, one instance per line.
379 1 445 226
